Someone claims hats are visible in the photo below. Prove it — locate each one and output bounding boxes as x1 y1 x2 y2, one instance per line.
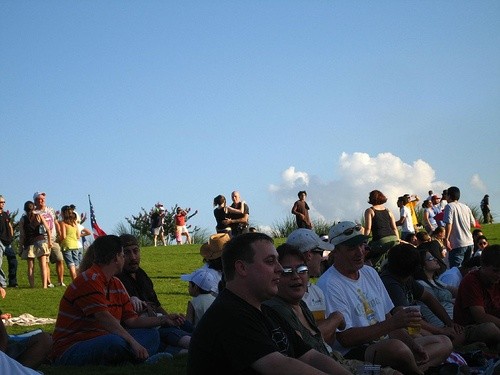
181 268 218 294
286 228 335 254
200 234 231 260
330 221 372 249
118 234 140 248
33 192 46 201
159 207 164 211
431 195 440 200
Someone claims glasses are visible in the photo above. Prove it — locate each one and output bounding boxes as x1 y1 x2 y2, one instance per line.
306 249 323 258
407 197 410 199
280 264 310 277
478 242 487 246
329 223 362 244
424 256 436 262
398 199 401 203
178 210 182 211
0 201 5 203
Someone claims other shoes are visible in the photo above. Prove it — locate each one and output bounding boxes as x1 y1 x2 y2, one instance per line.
48 283 66 288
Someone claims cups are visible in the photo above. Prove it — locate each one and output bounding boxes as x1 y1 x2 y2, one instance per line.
403 305 421 335
309 307 326 324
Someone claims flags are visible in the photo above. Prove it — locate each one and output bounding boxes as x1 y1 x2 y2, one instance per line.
89 201 107 239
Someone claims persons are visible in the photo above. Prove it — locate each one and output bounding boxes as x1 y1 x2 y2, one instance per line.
188 232 354 375
480 195 491 224
0 287 54 375
200 233 231 274
259 243 403 374
315 222 471 375
53 234 196 369
18 201 54 288
175 207 192 244
285 227 346 348
33 191 67 288
213 191 256 240
55 205 91 281
150 203 168 247
377 226 500 352
395 187 476 270
291 190 313 229
180 267 221 330
363 190 400 273
0 195 23 288
